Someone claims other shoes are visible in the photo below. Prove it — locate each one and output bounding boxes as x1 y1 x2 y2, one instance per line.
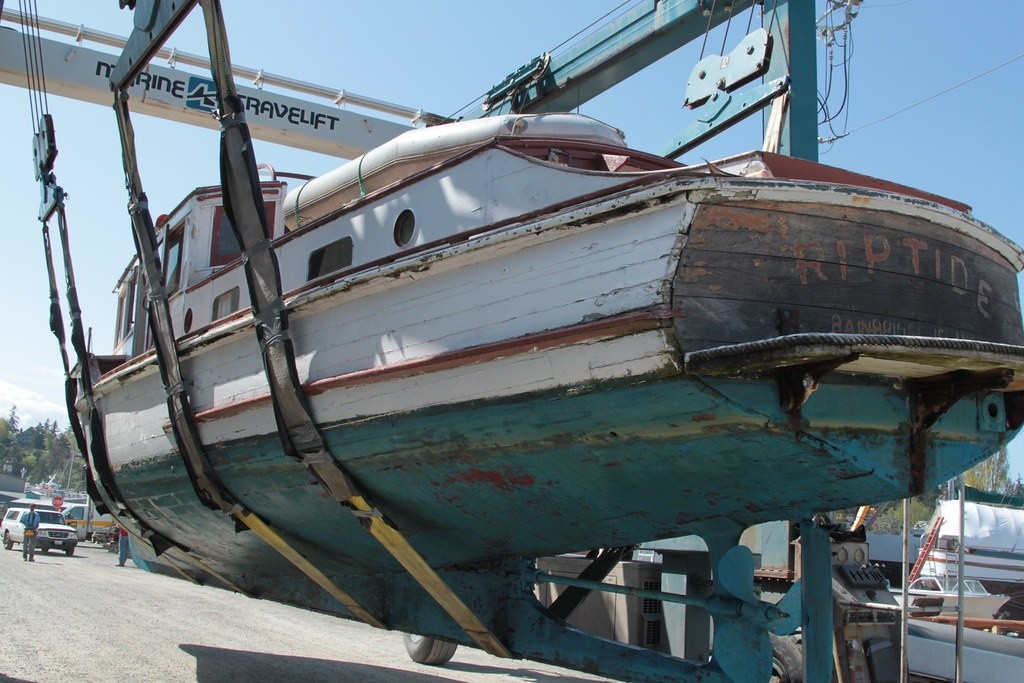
24 557 27 561
29 557 35 562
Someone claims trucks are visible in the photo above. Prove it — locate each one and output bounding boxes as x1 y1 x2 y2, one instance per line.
60 505 120 543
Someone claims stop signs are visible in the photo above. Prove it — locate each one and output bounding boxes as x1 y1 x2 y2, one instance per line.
52 497 63 508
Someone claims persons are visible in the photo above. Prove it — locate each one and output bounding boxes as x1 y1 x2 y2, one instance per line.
115 524 129 566
20 503 39 563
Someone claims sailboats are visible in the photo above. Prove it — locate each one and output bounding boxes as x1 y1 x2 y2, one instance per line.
31 454 87 504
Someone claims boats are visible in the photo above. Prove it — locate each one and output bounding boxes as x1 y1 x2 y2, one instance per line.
76 110 1023 683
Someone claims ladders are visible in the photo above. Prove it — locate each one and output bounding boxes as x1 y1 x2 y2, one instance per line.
908 516 945 588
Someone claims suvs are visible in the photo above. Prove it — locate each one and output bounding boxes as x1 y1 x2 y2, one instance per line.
0 508 78 557
